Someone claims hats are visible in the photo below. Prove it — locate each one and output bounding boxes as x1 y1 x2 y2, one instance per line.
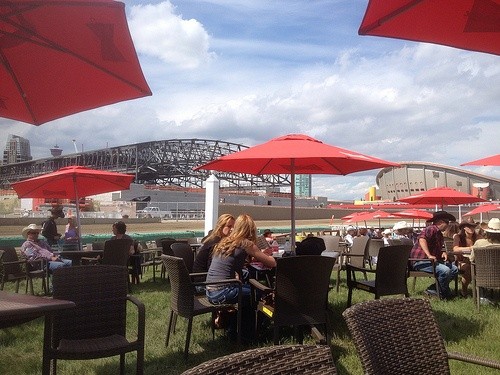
392 221 411 230
459 221 477 229
370 227 375 231
47 205 65 218
480 217 500 233
21 224 44 239
345 226 354 234
425 211 456 223
262 229 274 236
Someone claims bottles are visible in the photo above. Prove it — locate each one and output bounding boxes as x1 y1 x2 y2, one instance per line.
284 240 291 255
272 239 279 255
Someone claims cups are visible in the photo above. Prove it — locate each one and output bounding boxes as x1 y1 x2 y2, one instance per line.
86 244 93 251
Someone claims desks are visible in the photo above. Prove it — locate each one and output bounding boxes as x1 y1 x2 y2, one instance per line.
249 262 274 302
56 249 104 258
0 290 77 375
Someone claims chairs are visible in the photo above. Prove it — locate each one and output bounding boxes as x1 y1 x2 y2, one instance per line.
0 228 500 375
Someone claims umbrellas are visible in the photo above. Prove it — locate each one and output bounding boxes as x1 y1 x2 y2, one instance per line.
358 0 500 58
0 0 152 125
397 186 491 211
462 204 500 219
342 209 433 231
461 154 500 167
10 165 135 252
194 133 401 248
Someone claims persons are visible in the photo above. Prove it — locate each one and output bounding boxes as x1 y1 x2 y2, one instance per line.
193 213 250 328
97 220 134 265
346 221 411 265
410 211 458 298
41 206 65 247
470 217 500 303
263 229 274 246
20 225 72 273
453 218 482 297
207 215 275 335
64 216 79 243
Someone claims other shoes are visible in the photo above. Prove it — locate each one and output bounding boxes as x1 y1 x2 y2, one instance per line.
461 289 467 298
241 335 260 345
211 312 224 329
424 288 443 298
441 293 455 300
